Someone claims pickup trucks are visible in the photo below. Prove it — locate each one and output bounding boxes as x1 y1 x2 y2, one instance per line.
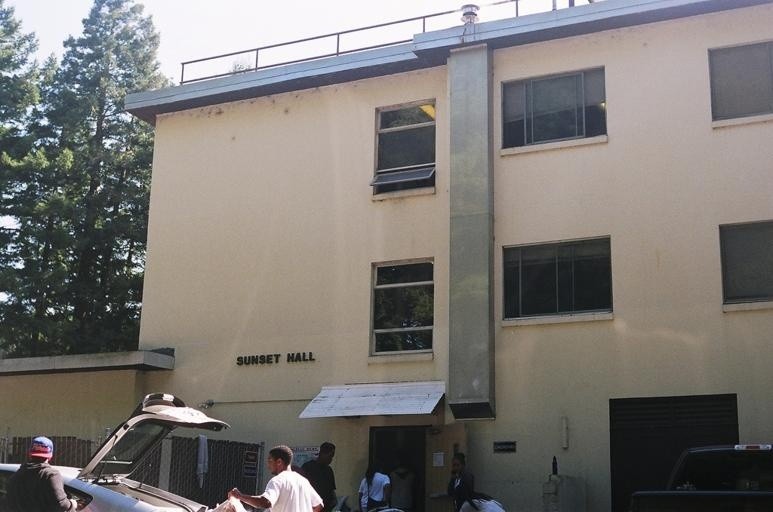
630 443 772 512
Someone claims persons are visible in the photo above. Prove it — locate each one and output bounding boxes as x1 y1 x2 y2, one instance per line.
5 434 76 512
453 484 505 512
226 444 324 512
445 451 474 511
359 457 416 512
300 443 338 512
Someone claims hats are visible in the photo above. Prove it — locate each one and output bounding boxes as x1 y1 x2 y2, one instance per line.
29 437 53 458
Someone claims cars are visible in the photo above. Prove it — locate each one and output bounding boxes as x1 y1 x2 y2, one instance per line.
0 392 231 512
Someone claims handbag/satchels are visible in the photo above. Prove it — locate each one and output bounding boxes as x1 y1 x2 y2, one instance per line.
367 496 383 512
